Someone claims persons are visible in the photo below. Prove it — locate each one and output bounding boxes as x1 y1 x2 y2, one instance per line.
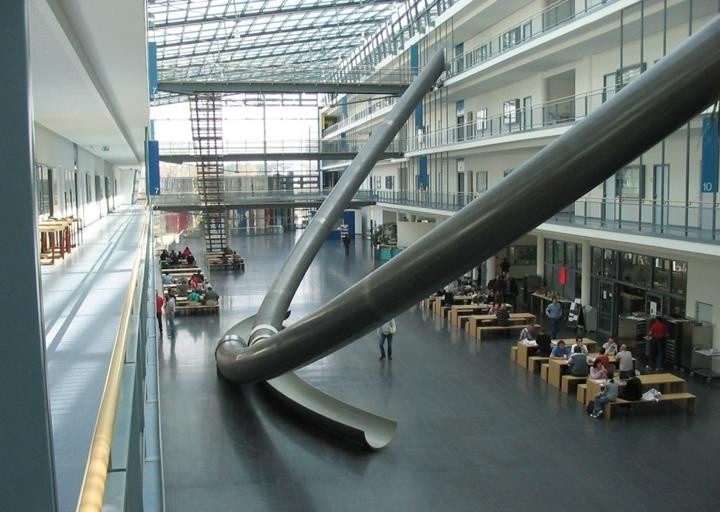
645 315 670 372
435 255 643 419
376 318 397 361
155 245 241 340
342 234 351 256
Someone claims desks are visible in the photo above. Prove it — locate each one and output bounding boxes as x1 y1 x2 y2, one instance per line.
531 292 570 314
38 216 82 265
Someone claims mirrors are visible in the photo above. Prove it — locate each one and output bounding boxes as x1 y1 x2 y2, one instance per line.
507 245 536 265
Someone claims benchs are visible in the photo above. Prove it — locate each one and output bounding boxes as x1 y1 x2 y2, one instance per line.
418 296 541 340
510 337 697 420
159 254 244 317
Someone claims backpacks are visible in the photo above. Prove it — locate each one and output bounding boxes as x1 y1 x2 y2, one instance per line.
625 377 643 401
